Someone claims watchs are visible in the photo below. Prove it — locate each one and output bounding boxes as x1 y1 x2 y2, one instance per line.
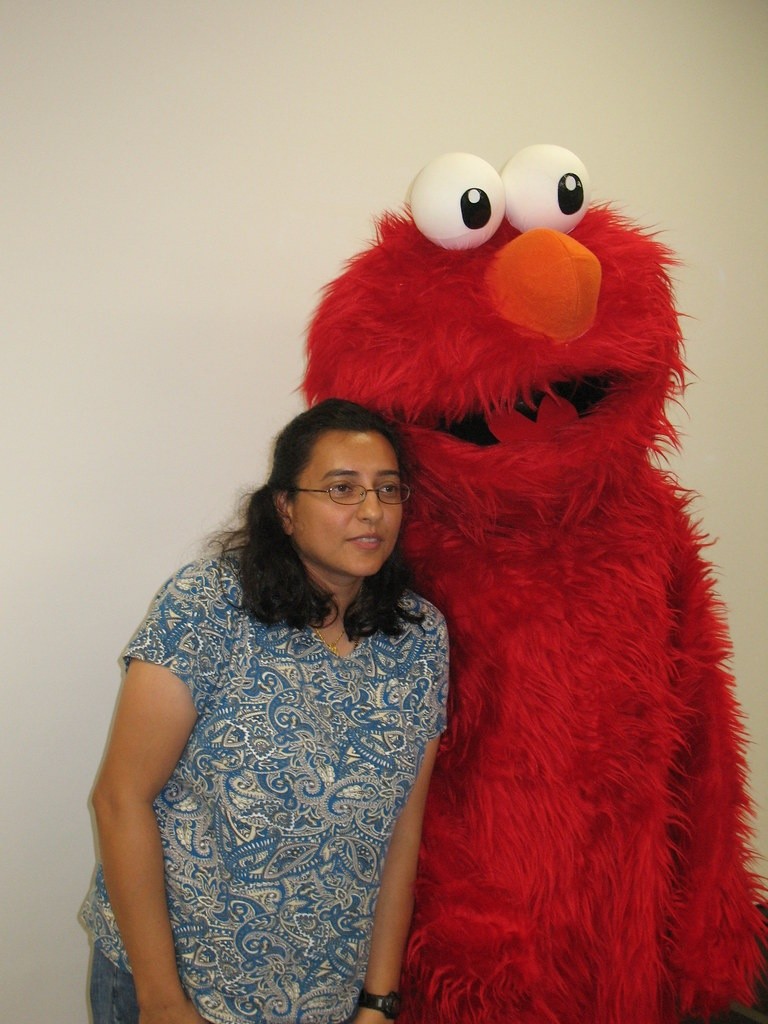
358 988 401 1019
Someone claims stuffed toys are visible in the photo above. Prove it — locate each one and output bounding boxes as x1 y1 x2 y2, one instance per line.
300 146 768 1024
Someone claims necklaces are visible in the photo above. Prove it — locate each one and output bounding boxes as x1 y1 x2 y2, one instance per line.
314 625 345 658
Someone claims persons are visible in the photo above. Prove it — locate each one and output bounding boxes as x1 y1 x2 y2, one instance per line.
77 398 451 1024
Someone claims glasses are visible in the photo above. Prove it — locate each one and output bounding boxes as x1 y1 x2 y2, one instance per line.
293 480 412 506
